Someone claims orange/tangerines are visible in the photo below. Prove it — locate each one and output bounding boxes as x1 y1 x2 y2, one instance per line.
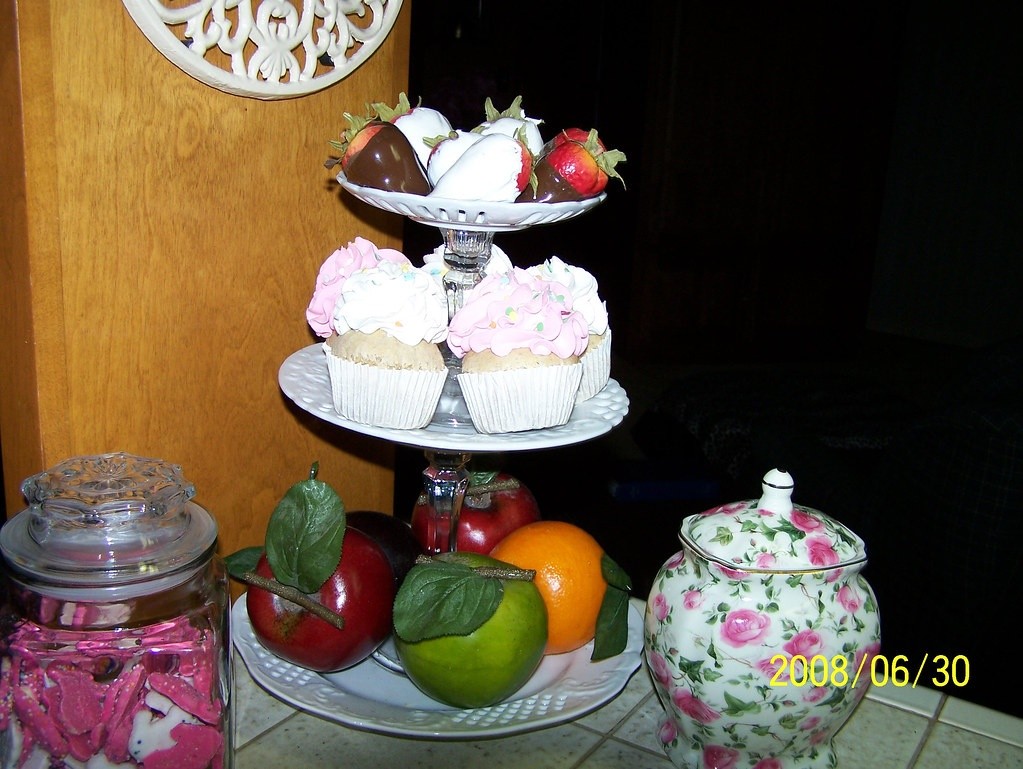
490 521 607 654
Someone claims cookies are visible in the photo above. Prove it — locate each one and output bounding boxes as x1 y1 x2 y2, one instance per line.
0 615 224 769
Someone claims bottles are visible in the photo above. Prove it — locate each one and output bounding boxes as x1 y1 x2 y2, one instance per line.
0 450 237 769
643 471 882 768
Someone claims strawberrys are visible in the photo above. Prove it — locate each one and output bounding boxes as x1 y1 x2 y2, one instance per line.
322 95 626 204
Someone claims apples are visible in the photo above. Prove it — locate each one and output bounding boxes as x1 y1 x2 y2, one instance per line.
246 469 547 706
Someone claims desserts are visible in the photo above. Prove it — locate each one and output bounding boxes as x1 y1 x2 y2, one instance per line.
306 237 610 437
